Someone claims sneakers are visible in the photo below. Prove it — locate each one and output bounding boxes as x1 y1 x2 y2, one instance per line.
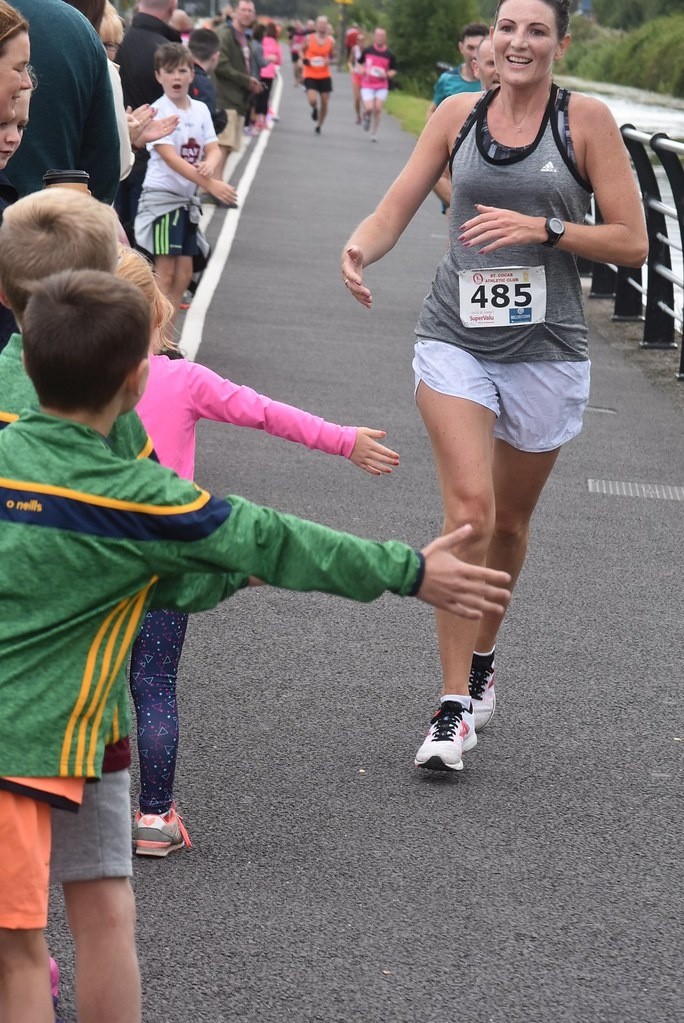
414 697 479 771
133 806 192 858
465 644 496 731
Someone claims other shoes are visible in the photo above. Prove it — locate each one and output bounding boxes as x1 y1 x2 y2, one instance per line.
254 120 268 129
315 126 321 134
312 106 319 122
160 347 183 361
244 127 259 137
363 114 370 131
180 291 190 309
198 192 236 208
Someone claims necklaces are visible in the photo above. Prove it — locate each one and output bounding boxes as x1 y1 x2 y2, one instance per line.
514 97 550 133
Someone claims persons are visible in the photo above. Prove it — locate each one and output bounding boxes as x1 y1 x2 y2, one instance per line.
0 0 515 1023
339 0 649 772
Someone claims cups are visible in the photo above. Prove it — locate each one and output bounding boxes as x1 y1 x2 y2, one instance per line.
42 168 88 191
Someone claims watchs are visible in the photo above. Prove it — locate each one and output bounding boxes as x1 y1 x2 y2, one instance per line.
544 215 566 248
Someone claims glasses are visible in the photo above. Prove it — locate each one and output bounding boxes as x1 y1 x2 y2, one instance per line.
100 41 124 51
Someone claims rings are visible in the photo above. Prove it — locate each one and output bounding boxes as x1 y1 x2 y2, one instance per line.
163 128 168 131
345 279 349 288
